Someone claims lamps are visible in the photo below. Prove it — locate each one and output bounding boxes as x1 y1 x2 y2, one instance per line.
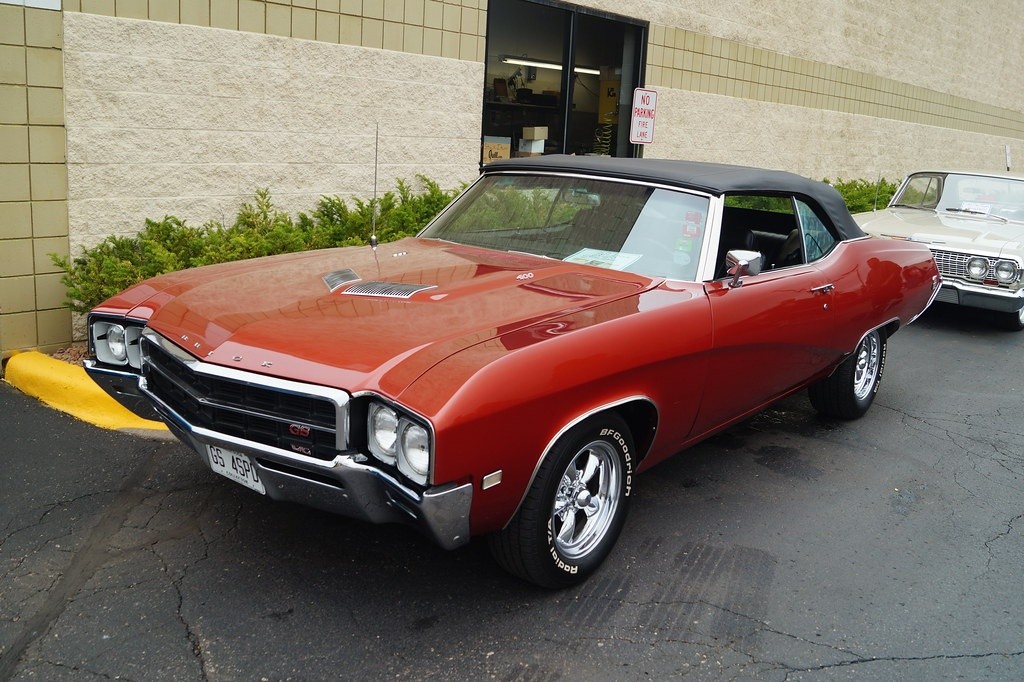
497 55 601 75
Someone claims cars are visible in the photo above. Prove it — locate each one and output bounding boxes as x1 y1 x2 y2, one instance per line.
843 170 1024 331
86 155 941 593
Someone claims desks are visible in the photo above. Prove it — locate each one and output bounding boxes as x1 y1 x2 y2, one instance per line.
483 100 561 139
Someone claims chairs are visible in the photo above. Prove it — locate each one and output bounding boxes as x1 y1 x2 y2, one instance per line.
714 219 756 280
569 206 640 255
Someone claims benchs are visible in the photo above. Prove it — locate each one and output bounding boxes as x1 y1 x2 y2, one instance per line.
748 230 789 258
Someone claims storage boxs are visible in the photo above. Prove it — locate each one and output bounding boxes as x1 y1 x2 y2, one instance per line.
518 139 544 153
522 126 549 139
518 152 541 159
